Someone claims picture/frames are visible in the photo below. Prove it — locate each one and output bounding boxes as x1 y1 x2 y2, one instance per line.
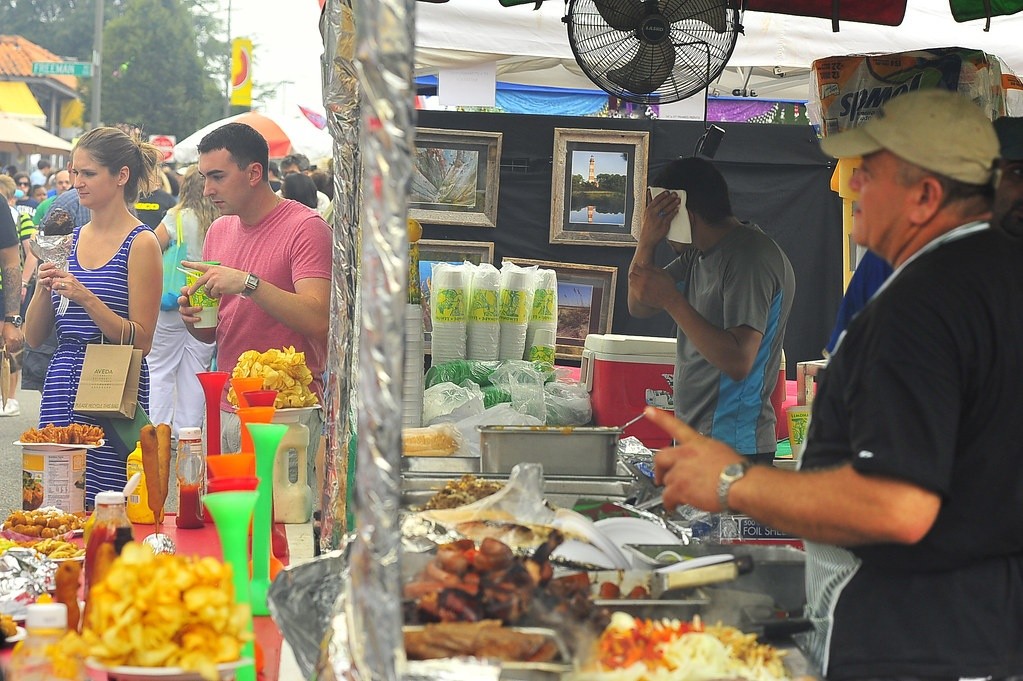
549 127 650 247
406 239 495 354
501 257 618 361
407 126 503 227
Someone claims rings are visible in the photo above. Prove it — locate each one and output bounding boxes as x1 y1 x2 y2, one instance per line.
61 283 65 290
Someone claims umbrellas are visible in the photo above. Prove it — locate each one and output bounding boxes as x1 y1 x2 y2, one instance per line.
0 114 74 158
172 105 332 165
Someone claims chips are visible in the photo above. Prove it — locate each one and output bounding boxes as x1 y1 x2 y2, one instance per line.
42 542 257 681
226 346 318 409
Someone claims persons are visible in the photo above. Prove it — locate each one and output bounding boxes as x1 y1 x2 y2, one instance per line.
0 120 332 517
646 96 1023 681
627 155 795 471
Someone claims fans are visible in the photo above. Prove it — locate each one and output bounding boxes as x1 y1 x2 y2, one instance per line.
567 0 740 104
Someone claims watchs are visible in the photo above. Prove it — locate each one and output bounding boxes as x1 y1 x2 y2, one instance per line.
3 315 23 329
241 272 259 298
717 459 766 514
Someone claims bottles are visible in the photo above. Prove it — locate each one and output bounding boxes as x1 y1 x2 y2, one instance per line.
9 603 84 681
175 427 205 528
85 491 134 601
126 441 164 524
269 416 313 525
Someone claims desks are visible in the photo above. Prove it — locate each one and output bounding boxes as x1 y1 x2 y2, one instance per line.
0 510 315 681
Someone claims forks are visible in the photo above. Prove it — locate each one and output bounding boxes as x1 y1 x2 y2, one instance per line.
177 266 247 300
57 261 69 316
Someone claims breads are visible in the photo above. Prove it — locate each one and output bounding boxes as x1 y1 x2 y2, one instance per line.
403 433 459 457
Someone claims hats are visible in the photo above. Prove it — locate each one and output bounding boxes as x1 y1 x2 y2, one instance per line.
819 88 1000 185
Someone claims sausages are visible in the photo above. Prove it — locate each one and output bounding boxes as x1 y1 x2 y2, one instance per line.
155 423 172 507
140 424 162 512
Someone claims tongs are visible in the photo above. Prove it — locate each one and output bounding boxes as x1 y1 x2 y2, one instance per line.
651 555 755 594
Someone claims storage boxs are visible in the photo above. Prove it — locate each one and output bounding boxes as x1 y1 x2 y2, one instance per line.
471 418 626 480
577 326 786 455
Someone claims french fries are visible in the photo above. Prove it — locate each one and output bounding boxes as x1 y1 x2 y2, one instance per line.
30 538 87 561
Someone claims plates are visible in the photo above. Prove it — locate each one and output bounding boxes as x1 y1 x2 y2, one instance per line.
232 404 323 415
85 656 254 681
12 427 105 452
593 517 681 548
556 508 632 570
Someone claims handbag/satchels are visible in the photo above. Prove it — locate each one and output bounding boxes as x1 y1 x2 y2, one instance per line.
73 317 144 420
105 323 154 457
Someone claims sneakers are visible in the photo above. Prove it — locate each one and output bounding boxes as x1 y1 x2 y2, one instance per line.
0 398 20 417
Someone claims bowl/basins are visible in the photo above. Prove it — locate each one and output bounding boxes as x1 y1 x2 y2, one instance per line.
401 305 423 428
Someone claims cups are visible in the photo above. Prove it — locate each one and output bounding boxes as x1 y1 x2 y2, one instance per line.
786 405 811 460
432 268 468 365
523 270 557 360
528 329 554 381
498 270 534 360
185 262 221 328
467 272 499 361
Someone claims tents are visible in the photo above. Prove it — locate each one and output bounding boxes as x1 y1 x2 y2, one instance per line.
316 0 997 565
